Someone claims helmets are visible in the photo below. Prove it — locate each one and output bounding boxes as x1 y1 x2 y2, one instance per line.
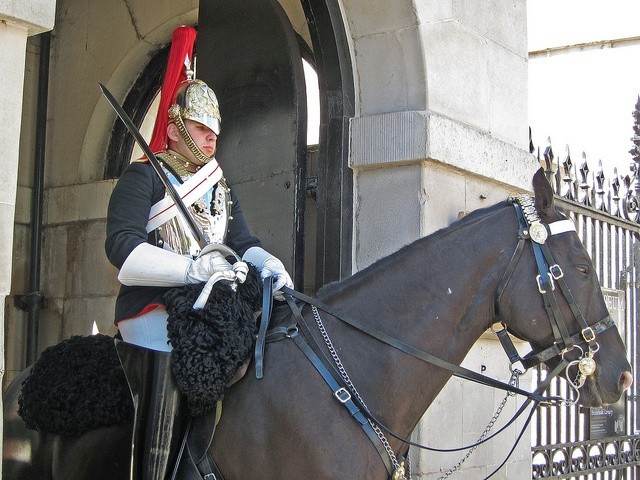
142 24 221 162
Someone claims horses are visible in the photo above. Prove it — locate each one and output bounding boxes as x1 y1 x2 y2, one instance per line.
51 164 633 475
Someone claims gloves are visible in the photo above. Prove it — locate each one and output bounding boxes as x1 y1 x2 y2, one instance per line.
118 242 232 287
242 247 294 301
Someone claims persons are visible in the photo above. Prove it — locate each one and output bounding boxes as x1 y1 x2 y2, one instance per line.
105 24 295 480
